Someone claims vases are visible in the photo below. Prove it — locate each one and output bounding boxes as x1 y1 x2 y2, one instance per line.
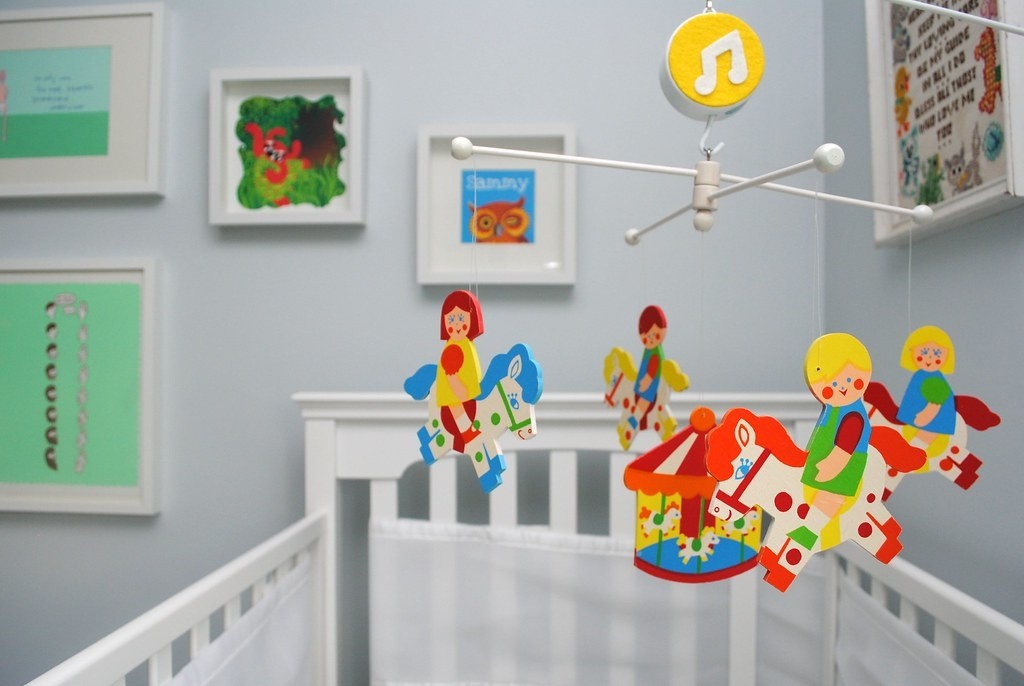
414 121 576 287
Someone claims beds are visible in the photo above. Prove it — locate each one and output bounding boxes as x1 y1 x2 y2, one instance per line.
23 387 1024 686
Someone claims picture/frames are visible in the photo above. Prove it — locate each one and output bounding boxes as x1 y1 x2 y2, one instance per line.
207 62 369 226
866 0 1024 249
0 259 163 517
0 3 165 197
